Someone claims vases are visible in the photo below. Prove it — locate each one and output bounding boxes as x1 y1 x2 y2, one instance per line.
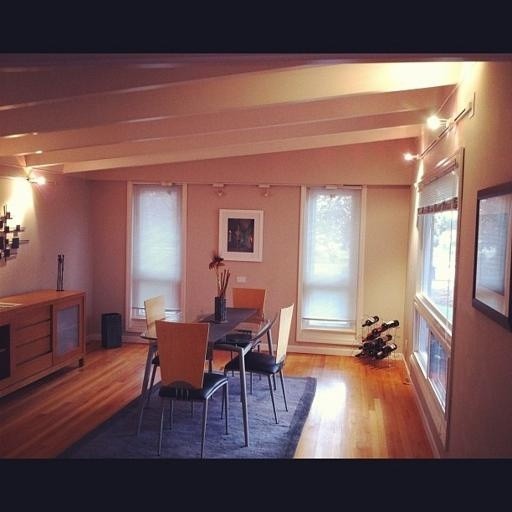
209 251 231 298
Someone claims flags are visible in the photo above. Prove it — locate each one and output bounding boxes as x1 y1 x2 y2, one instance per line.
214 297 229 325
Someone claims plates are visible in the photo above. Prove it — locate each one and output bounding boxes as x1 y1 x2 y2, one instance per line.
0 288 88 399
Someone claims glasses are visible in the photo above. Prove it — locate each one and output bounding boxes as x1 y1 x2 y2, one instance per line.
402 92 476 162
28 176 47 186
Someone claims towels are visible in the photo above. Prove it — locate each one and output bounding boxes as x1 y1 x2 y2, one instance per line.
101 313 121 348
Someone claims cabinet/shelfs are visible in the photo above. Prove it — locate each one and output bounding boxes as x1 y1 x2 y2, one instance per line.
355 316 400 360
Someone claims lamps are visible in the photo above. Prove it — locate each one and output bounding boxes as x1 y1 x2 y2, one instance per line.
220 303 296 425
230 287 267 380
154 319 231 460
144 294 214 417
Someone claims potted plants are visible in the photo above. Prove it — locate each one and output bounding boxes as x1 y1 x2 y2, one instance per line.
217 207 263 263
471 179 512 332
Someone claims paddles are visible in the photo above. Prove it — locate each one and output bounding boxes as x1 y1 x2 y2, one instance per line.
53 369 318 460
